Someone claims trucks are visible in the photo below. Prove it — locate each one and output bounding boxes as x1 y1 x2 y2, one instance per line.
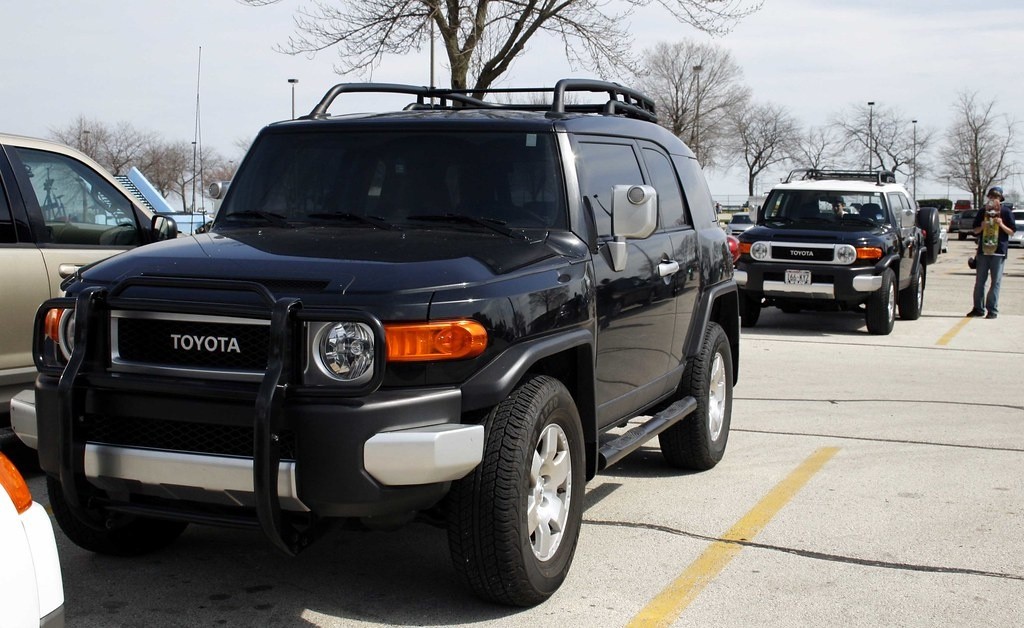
77 165 215 235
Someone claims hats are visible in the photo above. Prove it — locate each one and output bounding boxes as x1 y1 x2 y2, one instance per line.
987 187 1005 202
829 197 844 203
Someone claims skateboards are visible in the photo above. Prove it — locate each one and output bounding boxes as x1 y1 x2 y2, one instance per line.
982 200 999 254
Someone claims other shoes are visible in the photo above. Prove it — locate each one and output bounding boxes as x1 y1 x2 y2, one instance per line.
986 311 997 319
967 311 984 317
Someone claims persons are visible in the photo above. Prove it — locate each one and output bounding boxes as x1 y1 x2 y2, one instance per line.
831 198 848 217
966 186 1017 319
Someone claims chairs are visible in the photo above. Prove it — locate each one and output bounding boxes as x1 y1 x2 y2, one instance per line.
860 203 882 220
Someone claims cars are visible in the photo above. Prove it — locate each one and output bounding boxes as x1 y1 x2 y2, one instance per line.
947 214 962 233
956 209 980 241
953 199 974 213
939 223 948 254
724 211 775 239
1008 210 1024 248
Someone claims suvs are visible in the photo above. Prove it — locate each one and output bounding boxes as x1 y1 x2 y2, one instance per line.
730 168 941 337
0 132 192 415
10 77 748 610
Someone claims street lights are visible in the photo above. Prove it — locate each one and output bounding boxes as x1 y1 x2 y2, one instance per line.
691 65 704 164
287 78 298 119
190 141 197 212
868 101 876 204
912 119 917 201
81 130 92 223
